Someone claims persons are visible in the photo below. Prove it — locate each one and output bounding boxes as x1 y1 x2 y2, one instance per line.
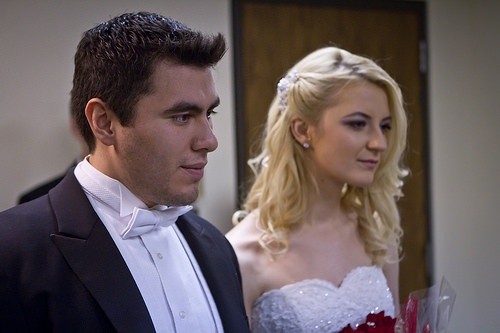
225 46 410 333
1 10 248 333
19 103 91 205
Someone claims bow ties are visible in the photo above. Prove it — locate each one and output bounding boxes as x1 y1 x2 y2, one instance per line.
74 163 194 240
